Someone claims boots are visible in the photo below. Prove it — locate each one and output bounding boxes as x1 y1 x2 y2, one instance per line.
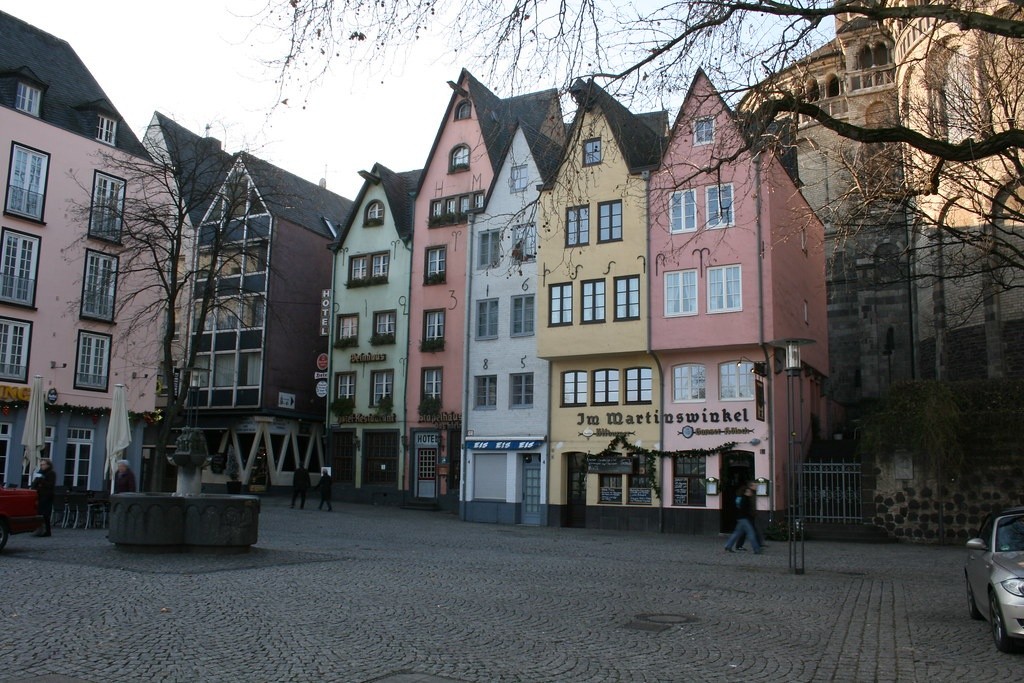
32 522 41 537
41 522 52 537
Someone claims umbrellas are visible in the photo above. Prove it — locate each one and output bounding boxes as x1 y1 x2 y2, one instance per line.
104 384 132 494
21 376 46 486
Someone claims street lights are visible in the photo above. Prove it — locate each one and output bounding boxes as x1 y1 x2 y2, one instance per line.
769 336 818 576
182 367 213 429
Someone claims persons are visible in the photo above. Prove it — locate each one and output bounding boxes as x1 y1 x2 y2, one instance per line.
314 470 332 511
106 460 135 538
735 481 770 551
725 487 763 554
290 463 311 509
33 460 55 537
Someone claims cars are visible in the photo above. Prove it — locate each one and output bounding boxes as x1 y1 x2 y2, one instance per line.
0 484 46 552
964 506 1024 654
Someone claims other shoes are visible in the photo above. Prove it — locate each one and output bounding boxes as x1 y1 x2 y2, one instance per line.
725 548 735 553
